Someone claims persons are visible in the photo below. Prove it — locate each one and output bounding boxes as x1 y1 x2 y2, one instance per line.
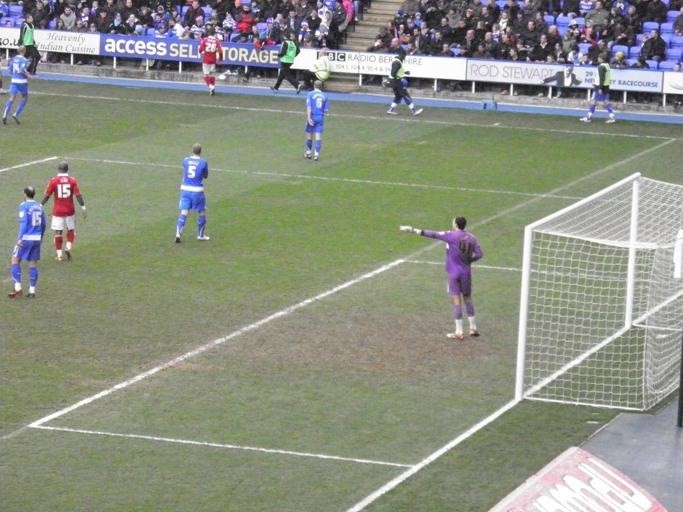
0 0 363 77
39 161 87 263
303 79 329 162
175 142 211 243
6 185 46 298
271 33 303 94
0 45 33 126
399 215 483 341
199 26 223 96
578 53 616 124
369 0 683 106
384 50 424 116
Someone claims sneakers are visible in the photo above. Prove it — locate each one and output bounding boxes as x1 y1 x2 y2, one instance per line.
7 289 22 298
210 87 215 96
54 248 73 263
304 151 311 158
197 234 211 241
446 332 464 340
469 327 481 335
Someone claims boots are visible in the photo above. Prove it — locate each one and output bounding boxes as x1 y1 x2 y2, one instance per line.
410 108 423 117
387 106 399 115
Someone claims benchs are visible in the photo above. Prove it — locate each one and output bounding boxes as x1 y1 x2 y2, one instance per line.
0 0 682 70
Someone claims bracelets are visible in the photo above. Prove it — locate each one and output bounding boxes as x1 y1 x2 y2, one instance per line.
79 205 86 211
39 203 44 207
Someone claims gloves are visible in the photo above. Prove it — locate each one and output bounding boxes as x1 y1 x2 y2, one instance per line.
400 225 413 233
